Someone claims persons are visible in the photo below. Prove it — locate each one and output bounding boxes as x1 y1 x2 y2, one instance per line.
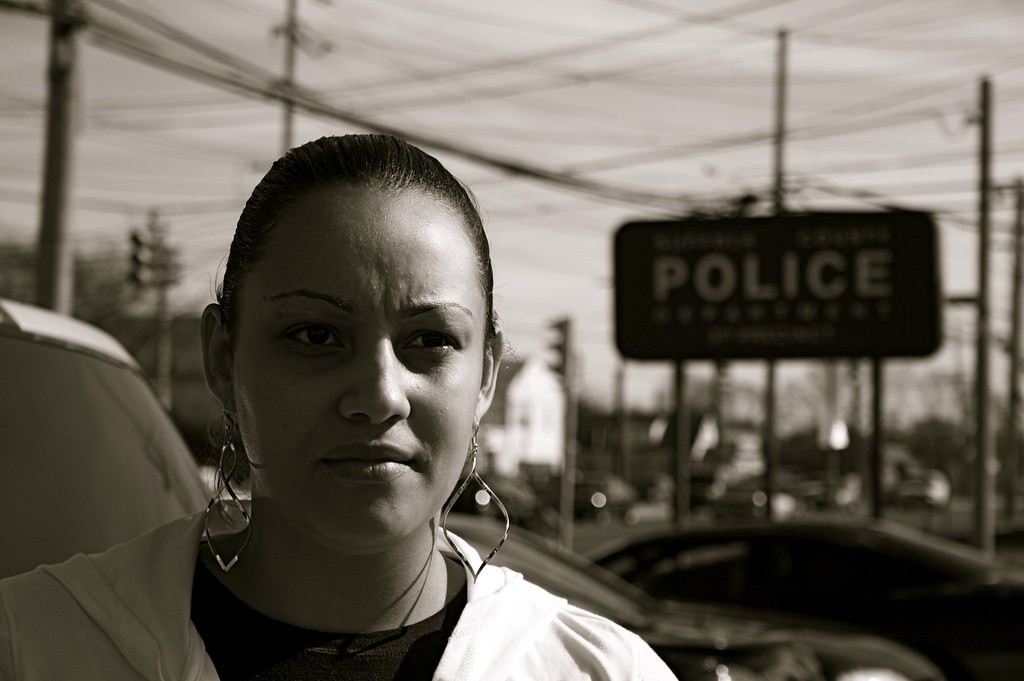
620 438 968 547
0 132 681 681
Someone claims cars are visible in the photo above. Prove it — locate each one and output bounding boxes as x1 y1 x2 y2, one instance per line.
584 511 1024 681
436 511 948 681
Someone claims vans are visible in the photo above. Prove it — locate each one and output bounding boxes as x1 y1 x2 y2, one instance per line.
0 299 220 582
